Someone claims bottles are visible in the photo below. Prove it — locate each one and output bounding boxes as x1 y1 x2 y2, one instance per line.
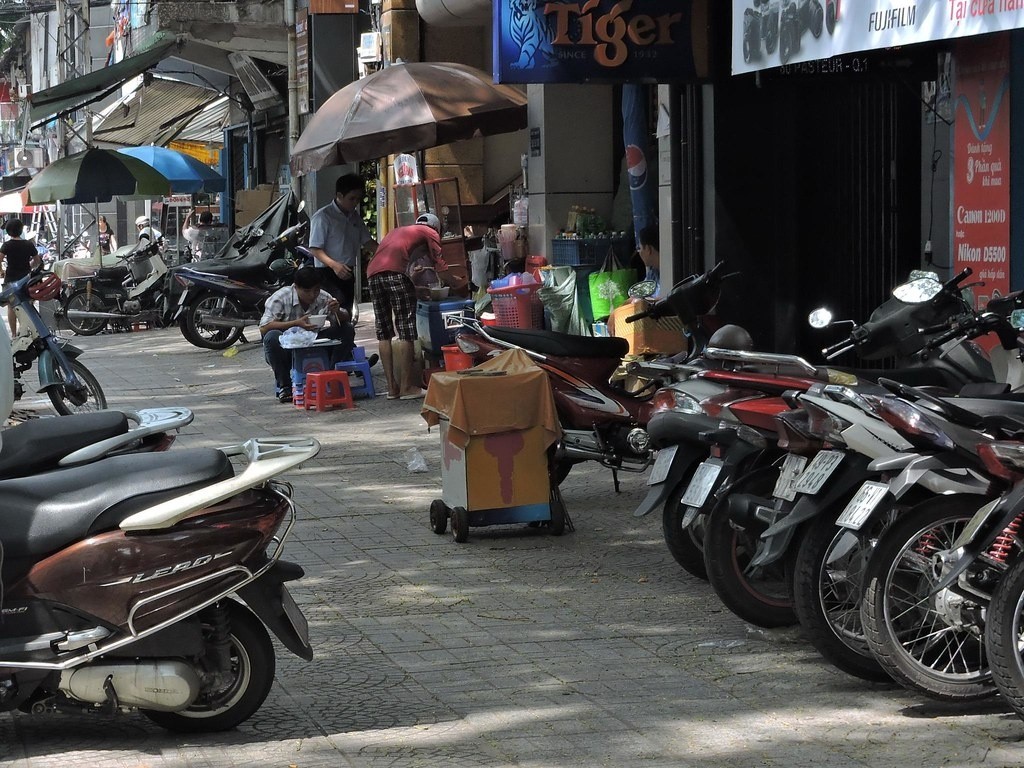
557 205 626 245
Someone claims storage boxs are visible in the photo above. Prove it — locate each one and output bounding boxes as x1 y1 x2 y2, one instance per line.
611 301 689 358
415 295 475 354
552 234 634 268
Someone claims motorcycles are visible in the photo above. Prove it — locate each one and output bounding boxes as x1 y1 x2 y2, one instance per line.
629 266 1024 723
154 189 315 351
0 408 313 739
62 233 171 336
442 258 744 495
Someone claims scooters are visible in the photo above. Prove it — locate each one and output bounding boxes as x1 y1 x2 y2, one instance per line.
0 263 108 419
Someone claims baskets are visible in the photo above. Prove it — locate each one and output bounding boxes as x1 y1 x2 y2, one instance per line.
487 283 543 330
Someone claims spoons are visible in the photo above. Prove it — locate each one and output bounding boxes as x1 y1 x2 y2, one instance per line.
319 305 328 315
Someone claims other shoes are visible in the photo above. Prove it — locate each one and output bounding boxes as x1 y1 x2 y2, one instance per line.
276 386 293 402
354 353 379 377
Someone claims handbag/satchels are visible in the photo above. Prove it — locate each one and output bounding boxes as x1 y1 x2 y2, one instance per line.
588 246 638 321
183 246 192 264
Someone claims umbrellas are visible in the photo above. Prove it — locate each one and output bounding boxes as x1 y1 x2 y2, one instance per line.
20 145 171 267
288 56 529 212
118 145 227 246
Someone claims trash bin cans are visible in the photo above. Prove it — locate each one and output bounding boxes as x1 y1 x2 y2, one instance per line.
486 282 544 330
480 312 496 326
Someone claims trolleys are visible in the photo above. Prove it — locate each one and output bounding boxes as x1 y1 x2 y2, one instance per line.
429 499 566 542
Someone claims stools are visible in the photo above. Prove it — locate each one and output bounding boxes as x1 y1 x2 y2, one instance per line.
290 348 376 415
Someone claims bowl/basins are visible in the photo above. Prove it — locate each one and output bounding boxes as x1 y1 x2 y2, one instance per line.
307 315 327 329
430 286 449 300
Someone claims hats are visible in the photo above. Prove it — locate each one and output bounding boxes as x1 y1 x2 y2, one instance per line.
416 214 441 234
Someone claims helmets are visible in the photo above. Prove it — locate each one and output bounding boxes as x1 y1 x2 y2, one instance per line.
135 216 149 226
709 325 753 349
27 272 61 300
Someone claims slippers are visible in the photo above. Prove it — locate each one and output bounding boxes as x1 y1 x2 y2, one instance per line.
387 394 400 399
399 388 427 399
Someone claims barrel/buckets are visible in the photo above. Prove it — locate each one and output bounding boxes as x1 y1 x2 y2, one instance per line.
442 345 472 372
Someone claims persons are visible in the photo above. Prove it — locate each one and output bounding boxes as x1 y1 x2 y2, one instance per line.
257 266 359 401
182 210 217 262
96 215 118 255
637 231 663 302
0 219 42 339
308 173 379 376
367 212 460 401
135 215 162 247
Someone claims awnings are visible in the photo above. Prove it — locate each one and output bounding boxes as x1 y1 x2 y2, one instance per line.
91 78 220 149
174 95 229 147
25 42 182 123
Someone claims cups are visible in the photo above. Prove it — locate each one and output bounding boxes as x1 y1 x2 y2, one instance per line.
353 347 365 362
